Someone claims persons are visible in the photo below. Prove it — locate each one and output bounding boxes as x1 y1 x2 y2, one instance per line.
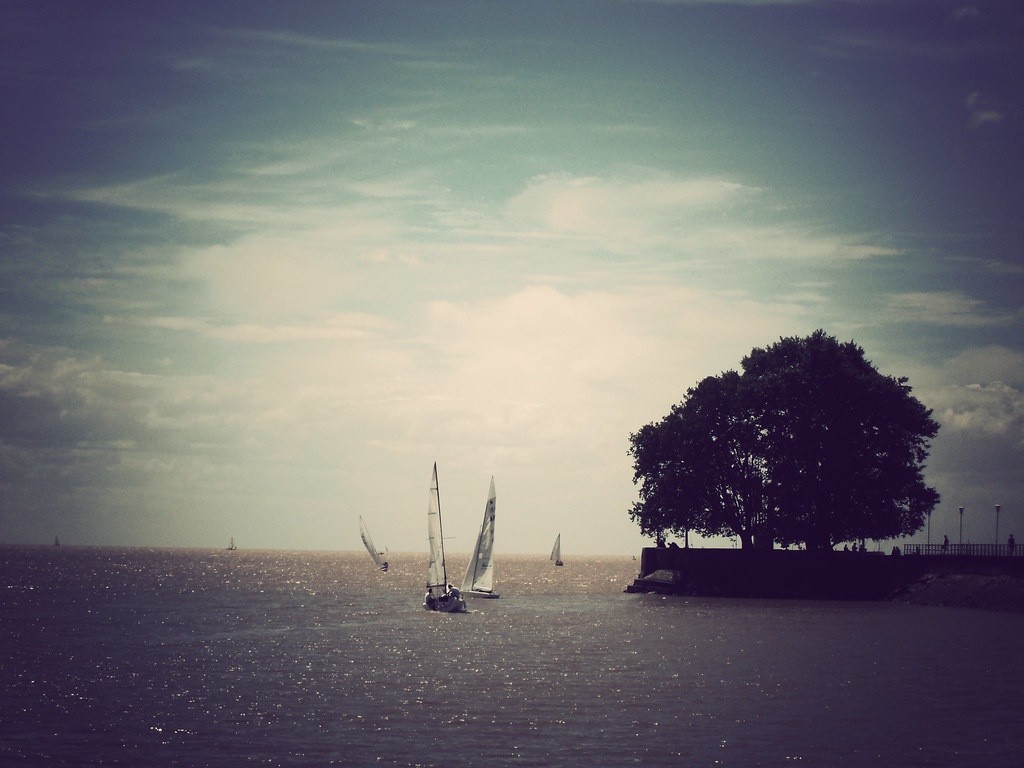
425 588 435 611
844 542 858 552
1008 534 1015 556
447 584 460 600
941 535 950 555
892 546 900 555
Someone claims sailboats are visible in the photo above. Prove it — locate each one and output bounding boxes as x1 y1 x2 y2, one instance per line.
224 536 236 551
549 533 564 566
460 474 502 599
422 461 468 612
357 514 389 572
